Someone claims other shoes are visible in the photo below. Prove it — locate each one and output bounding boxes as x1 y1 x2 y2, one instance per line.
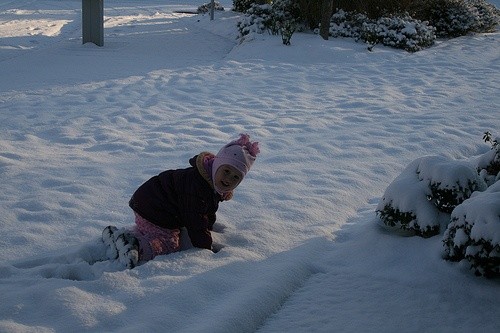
101 225 139 269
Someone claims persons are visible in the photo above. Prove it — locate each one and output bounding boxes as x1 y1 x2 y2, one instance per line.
101 133 262 268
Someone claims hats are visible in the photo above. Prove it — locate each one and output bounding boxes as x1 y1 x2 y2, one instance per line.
211 131 261 193
